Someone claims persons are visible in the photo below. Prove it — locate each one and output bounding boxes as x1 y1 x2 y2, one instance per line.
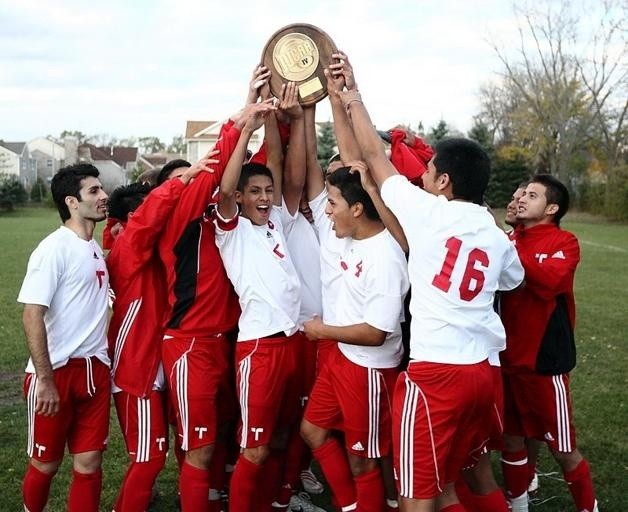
14 162 115 512
101 147 221 512
485 178 537 499
158 51 526 510
481 173 603 512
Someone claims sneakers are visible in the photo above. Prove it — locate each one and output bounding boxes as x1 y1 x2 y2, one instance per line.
301 469 324 493
287 494 327 512
